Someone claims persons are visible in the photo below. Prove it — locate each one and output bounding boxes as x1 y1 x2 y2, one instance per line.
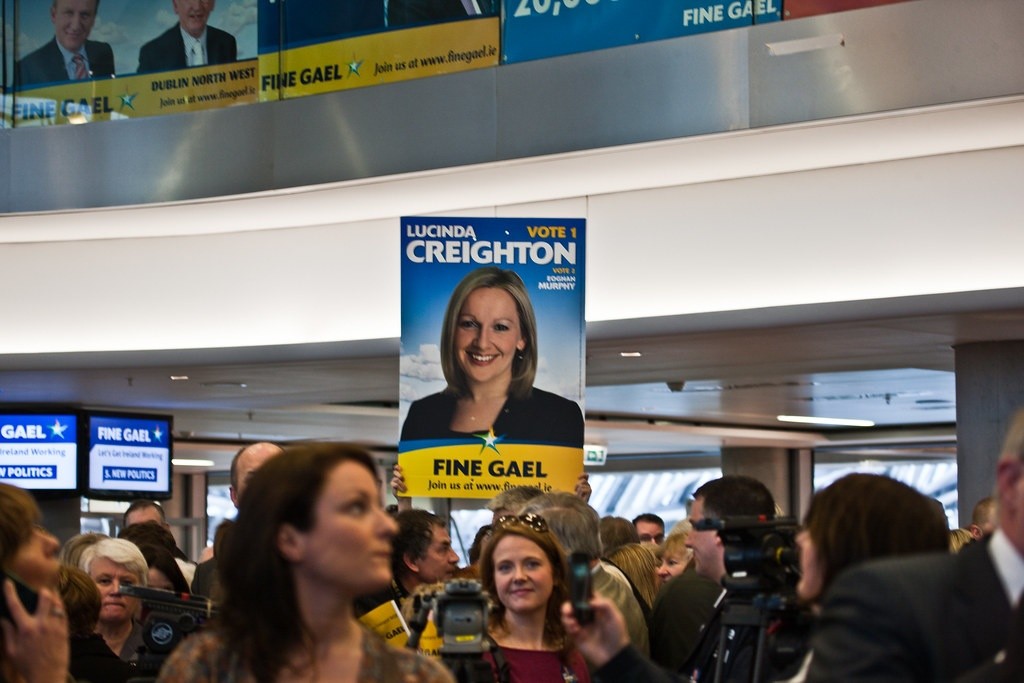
15 0 115 86
152 441 456 683
137 0 237 74
400 266 585 450
0 406 1024 683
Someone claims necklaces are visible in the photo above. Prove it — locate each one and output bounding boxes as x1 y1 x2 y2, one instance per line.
468 416 476 420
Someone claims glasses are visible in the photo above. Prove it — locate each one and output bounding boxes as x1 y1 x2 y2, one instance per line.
639 534 664 542
496 515 549 534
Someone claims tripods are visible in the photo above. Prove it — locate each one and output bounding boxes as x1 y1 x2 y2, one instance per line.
680 576 811 683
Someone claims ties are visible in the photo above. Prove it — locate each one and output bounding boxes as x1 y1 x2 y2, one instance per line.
192 40 205 66
72 55 89 80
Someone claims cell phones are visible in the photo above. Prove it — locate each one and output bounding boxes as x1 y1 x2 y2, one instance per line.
569 551 597 626
0 569 39 622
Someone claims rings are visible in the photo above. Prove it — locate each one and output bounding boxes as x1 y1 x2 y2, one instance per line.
51 608 66 618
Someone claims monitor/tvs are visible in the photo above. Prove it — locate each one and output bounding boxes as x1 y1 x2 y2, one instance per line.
0 408 173 503
